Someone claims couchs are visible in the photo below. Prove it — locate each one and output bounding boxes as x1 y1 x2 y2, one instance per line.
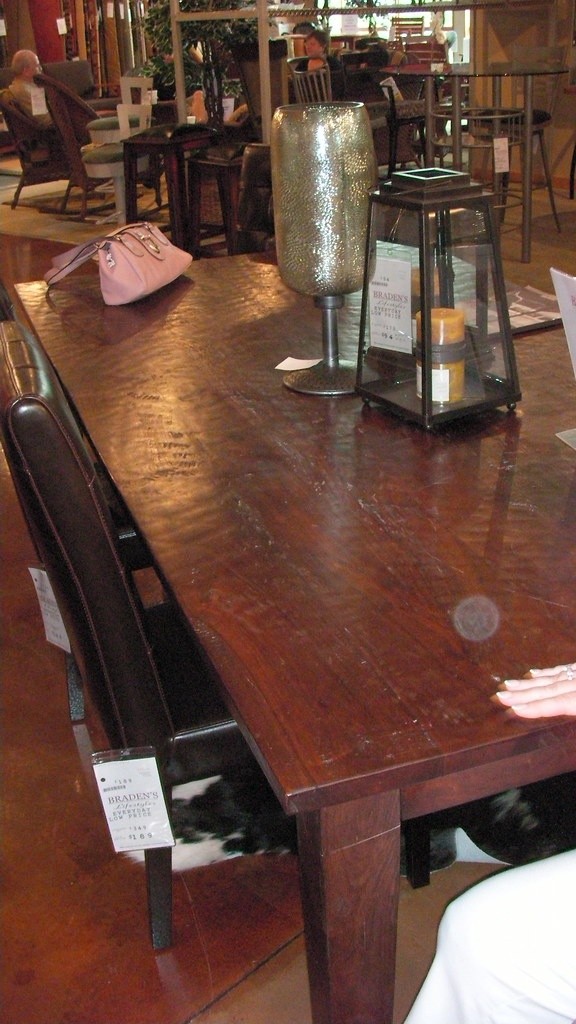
0 59 121 157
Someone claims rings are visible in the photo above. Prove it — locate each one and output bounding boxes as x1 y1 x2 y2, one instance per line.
567 668 573 680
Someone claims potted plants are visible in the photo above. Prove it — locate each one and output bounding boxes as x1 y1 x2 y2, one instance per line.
137 0 273 227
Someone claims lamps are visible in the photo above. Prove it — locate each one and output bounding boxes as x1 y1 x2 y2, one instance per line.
270 100 376 397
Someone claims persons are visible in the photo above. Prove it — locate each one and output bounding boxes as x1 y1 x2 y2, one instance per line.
9 49 114 192
407 663 576 1024
356 44 389 98
295 31 347 101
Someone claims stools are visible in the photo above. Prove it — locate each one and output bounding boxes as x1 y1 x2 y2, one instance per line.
185 141 251 257
122 124 222 247
473 109 561 234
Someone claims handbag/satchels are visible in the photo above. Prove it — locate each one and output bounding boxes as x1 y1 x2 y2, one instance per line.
44 221 192 305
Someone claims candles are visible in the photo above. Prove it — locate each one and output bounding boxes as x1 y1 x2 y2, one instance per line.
413 308 466 404
408 266 439 313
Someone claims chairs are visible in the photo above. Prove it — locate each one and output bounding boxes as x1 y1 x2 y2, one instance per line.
82 103 151 224
0 88 72 210
287 55 332 103
87 77 153 142
376 84 425 178
32 74 143 223
0 320 429 951
340 52 371 99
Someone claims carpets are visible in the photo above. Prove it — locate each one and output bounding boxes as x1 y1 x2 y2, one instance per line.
0 159 169 243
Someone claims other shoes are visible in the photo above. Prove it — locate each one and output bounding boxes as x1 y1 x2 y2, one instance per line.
143 164 165 188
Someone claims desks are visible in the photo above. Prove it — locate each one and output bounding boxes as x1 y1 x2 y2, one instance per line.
12 254 576 1024
380 61 569 265
